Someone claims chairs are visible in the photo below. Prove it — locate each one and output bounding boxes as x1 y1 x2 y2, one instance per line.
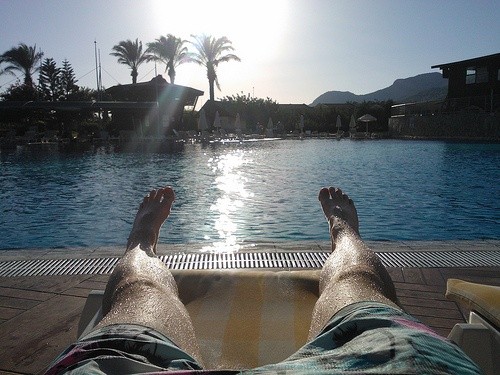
76 269 321 369
443 278 500 375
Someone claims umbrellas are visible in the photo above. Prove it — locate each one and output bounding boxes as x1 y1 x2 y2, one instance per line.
196 109 357 139
357 113 378 134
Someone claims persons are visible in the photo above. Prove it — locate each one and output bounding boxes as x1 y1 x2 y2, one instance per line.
42 186 484 375
